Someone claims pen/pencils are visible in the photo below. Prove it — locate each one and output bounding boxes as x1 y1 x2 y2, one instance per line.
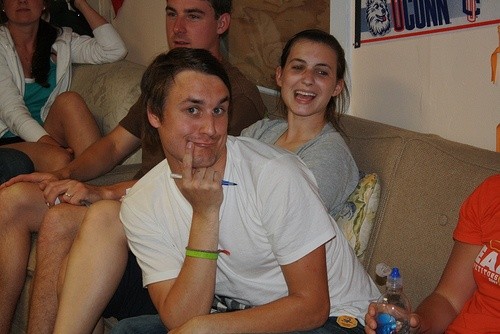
170 174 238 186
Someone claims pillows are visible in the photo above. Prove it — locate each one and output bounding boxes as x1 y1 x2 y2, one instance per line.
334 173 382 260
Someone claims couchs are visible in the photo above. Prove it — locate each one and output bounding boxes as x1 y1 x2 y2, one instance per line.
8 53 145 334
254 92 500 313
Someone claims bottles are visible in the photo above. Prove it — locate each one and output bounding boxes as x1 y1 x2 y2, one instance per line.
374 268 410 334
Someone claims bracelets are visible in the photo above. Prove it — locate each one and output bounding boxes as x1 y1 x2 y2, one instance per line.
185 247 219 260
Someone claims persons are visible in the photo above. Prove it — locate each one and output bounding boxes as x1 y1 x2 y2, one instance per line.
0 0 128 185
110 47 389 334
365 173 500 334
8 0 268 334
240 30 359 221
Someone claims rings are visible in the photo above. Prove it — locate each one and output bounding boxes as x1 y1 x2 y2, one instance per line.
64 192 71 196
